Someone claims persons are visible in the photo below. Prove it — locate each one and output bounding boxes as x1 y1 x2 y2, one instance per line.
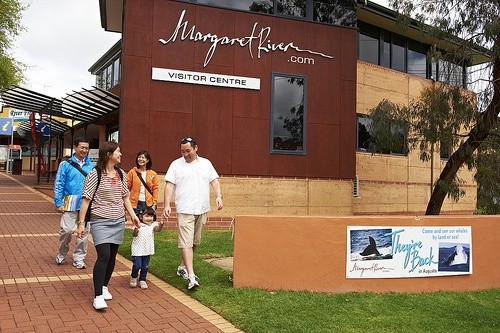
161 137 224 290
53 137 97 269
126 150 159 216
75 140 142 309
129 207 165 290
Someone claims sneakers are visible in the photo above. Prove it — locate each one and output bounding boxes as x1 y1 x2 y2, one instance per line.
188 275 200 289
176 266 190 280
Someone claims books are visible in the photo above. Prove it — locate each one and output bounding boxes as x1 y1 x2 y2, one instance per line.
55 194 78 211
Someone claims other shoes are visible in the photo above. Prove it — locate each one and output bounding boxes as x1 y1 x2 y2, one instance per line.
92 295 108 310
130 276 137 287
103 286 112 300
55 255 66 264
138 280 148 289
73 261 86 268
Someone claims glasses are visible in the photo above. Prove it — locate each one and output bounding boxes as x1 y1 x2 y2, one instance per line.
78 146 89 149
179 138 194 144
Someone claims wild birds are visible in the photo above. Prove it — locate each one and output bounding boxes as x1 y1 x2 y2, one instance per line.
444 252 458 267
359 236 382 257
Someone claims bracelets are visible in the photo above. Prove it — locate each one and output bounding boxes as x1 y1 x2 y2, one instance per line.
77 221 86 226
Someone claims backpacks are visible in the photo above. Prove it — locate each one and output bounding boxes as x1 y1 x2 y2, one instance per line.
85 167 123 222
